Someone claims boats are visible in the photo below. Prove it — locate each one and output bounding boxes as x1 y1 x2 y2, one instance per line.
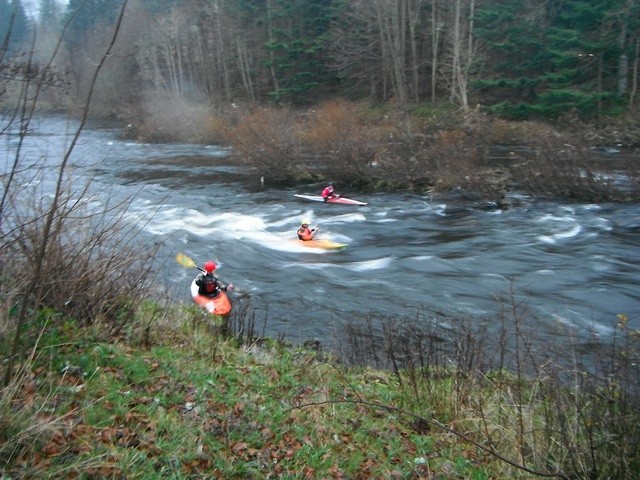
190 275 231 316
283 238 347 250
294 194 366 205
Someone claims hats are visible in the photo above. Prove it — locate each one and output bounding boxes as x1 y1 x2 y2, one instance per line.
302 219 313 225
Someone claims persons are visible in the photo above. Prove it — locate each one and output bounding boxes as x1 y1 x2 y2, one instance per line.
196 262 233 297
322 181 336 203
297 218 318 240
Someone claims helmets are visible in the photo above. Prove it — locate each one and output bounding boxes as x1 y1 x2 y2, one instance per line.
204 262 218 274
